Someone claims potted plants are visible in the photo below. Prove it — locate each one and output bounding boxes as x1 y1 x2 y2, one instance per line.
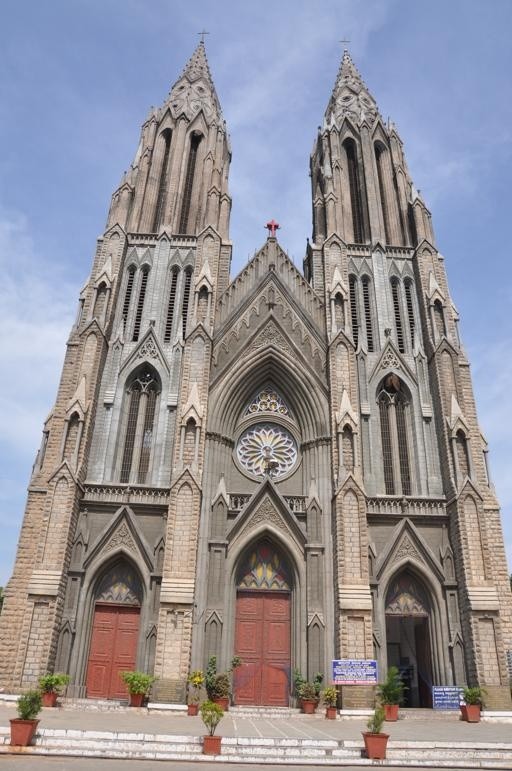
361 711 389 760
291 670 323 715
200 700 223 756
118 671 155 707
373 666 407 721
39 673 72 707
458 688 487 724
11 692 42 745
187 671 207 717
321 687 339 719
209 673 229 711
